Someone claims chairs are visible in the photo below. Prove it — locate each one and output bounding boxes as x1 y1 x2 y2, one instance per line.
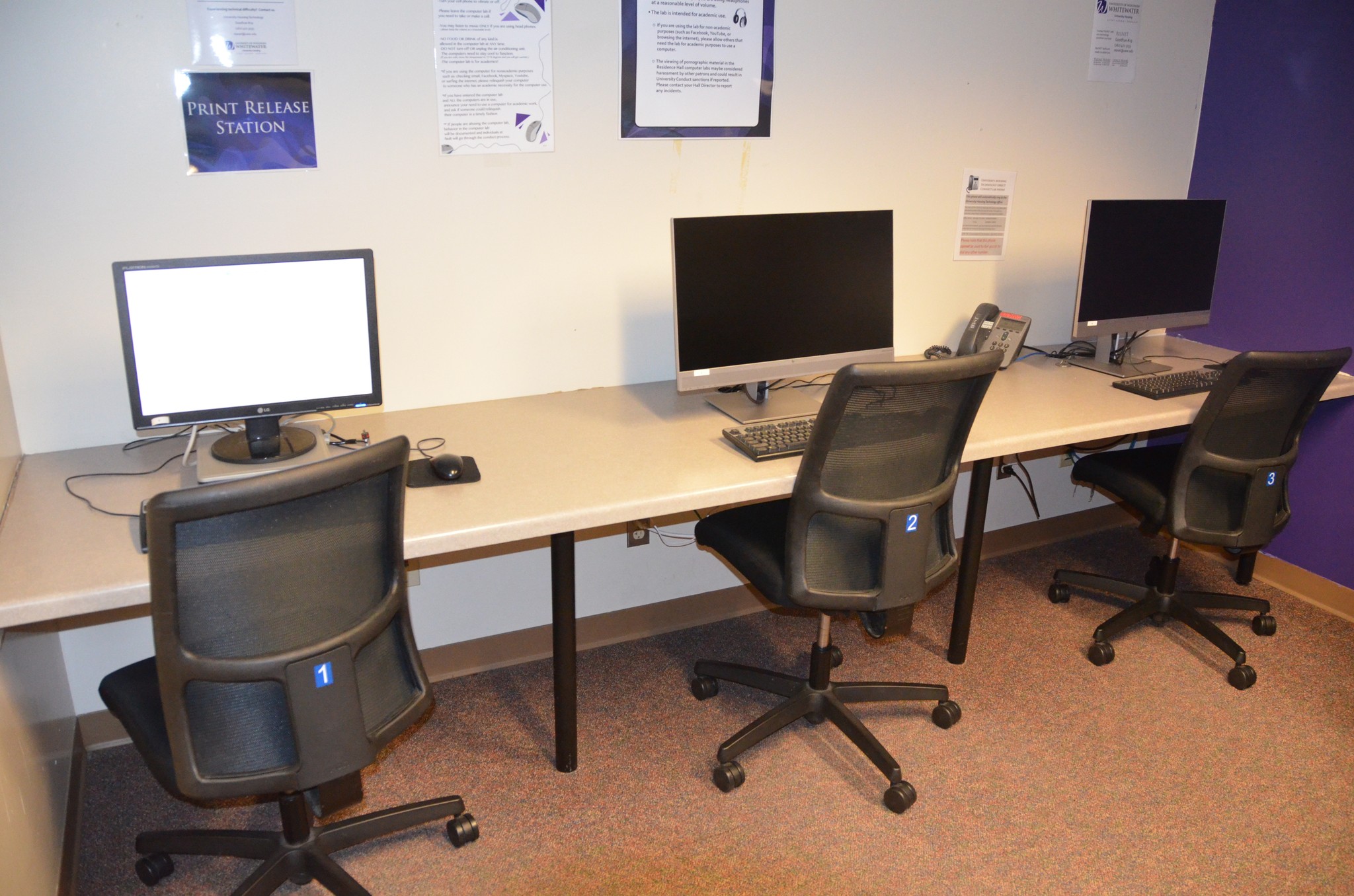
1044 340 1354 689
685 349 1010 810
98 435 482 896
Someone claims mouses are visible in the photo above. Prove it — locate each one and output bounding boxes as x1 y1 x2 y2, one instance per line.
429 453 463 480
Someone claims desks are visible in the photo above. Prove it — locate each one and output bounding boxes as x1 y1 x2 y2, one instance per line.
2 334 1354 775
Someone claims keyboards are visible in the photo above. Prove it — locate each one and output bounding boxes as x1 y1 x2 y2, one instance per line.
1112 364 1223 401
722 411 863 462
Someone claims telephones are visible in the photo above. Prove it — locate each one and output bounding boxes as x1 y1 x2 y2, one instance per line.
953 302 1033 369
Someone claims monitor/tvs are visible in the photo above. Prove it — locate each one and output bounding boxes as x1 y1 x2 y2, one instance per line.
112 247 382 431
1071 198 1228 340
670 209 896 392
